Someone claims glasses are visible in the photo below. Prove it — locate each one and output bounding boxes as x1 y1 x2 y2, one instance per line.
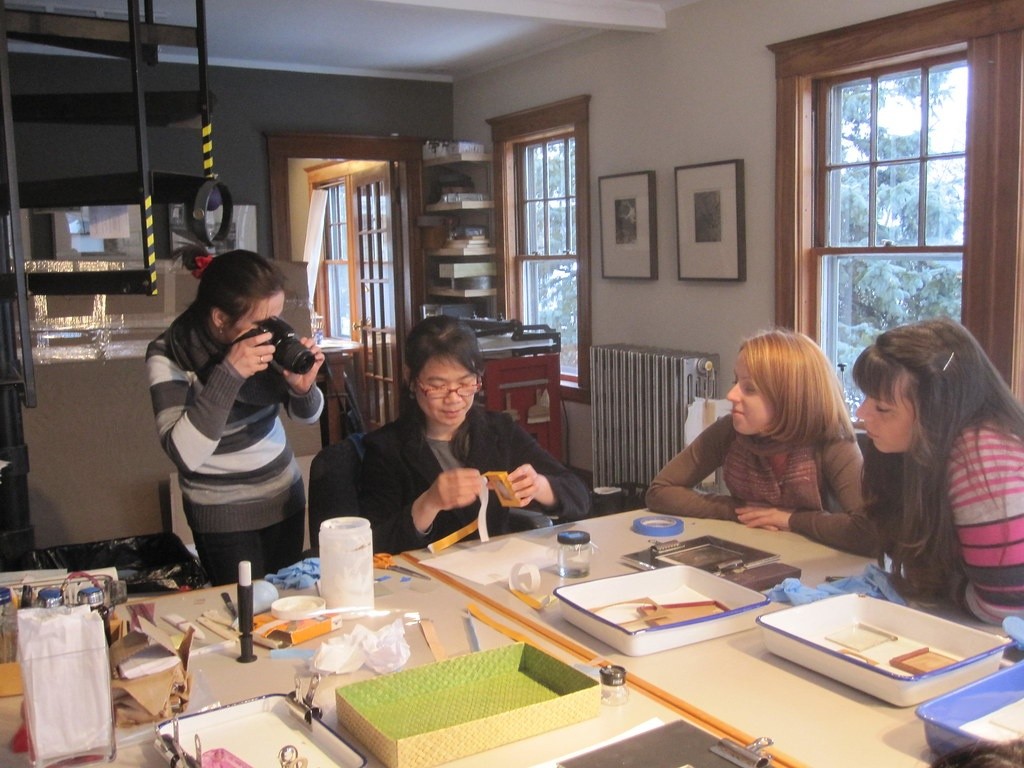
414 374 482 400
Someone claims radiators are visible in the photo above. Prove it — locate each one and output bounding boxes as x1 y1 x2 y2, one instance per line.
589 344 721 491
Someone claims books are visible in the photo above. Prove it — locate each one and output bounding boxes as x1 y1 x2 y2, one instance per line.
445 235 490 248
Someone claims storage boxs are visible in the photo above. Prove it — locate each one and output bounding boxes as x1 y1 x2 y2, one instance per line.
336 642 600 768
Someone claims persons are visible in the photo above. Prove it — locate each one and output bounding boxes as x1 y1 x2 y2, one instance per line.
646 331 880 558
144 242 325 588
854 317 1024 626
356 315 590 556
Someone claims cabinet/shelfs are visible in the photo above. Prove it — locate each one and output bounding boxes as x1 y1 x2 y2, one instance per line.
475 335 562 465
423 154 498 297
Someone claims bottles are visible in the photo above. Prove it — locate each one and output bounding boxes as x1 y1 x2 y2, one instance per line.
1 584 113 667
557 530 593 578
318 516 375 610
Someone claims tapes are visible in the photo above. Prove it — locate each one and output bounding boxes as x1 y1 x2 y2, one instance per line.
633 515 684 537
270 595 326 620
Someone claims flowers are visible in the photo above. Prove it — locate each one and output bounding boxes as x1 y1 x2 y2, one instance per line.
193 256 213 278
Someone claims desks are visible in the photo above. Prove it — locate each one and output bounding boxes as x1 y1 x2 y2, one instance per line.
316 339 363 450
1 510 1010 768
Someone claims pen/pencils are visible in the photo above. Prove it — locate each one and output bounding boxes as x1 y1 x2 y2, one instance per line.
826 576 848 581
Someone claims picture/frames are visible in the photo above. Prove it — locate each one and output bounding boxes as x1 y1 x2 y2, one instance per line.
598 170 659 279
168 201 262 259
675 159 747 282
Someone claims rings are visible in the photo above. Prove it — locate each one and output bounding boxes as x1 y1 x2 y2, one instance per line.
260 355 263 363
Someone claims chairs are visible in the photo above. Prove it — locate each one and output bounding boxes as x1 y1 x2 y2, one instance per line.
308 433 365 549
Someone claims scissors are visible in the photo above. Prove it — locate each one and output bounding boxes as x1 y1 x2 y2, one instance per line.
373 552 431 582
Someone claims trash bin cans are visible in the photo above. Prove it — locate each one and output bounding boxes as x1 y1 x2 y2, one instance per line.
28 531 208 594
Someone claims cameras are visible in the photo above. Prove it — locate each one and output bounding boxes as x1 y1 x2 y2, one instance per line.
237 315 316 374
